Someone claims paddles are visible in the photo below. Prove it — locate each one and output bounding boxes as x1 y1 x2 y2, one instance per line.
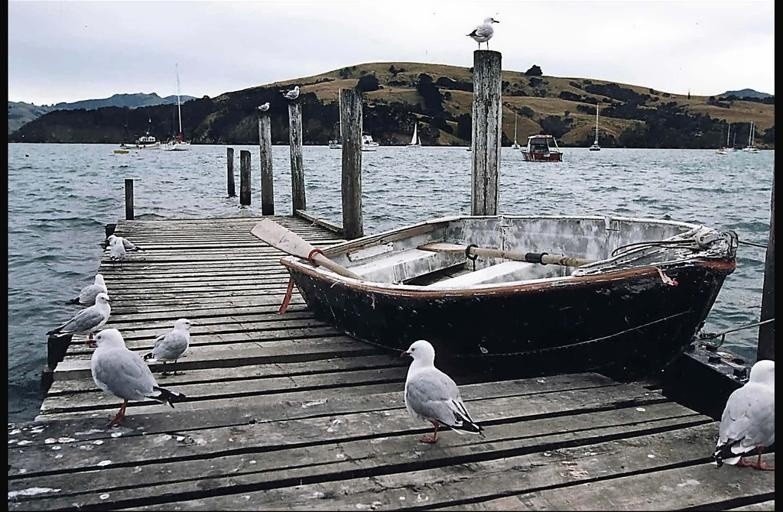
419 241 594 268
250 217 367 280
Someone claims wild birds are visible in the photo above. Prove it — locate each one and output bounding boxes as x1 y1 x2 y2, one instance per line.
399 340 485 444
86 328 185 429
65 274 108 306
141 318 197 376
105 234 145 262
713 360 775 471
466 15 499 51
45 293 111 349
284 86 300 99
256 102 271 112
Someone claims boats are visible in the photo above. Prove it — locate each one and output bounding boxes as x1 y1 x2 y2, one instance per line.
328 134 379 151
520 134 562 161
279 214 737 381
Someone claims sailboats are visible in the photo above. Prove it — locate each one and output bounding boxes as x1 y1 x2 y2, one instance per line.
715 120 759 154
134 118 160 148
161 73 189 150
405 122 422 147
588 103 600 150
